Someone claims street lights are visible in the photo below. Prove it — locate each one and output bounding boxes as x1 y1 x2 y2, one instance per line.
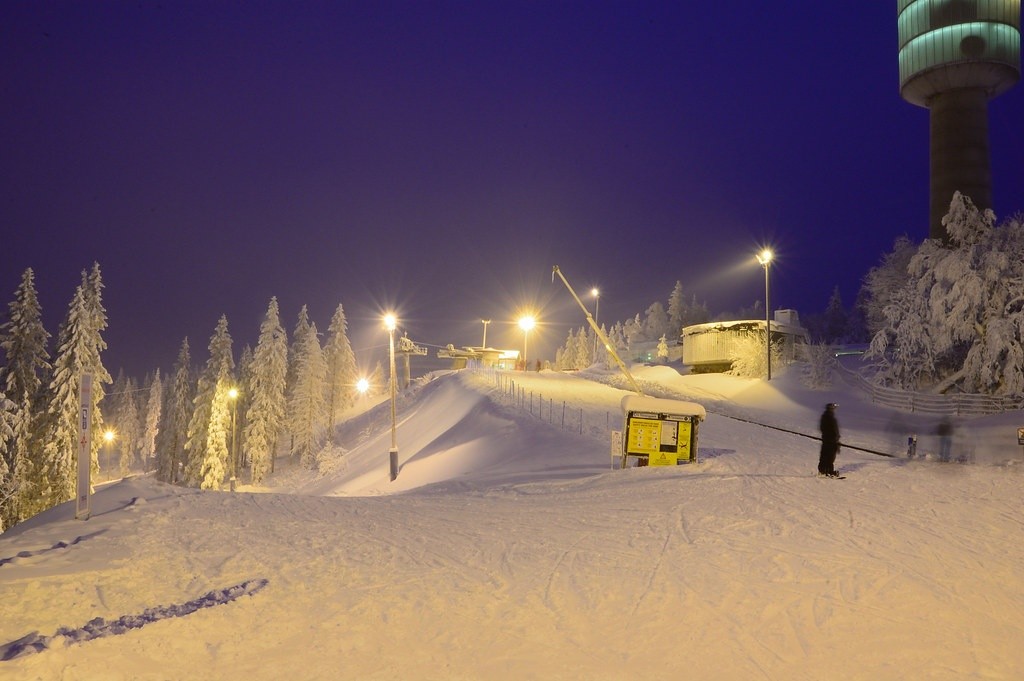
593 289 599 365
229 390 238 493
523 320 531 373
762 249 771 380
105 432 112 482
384 311 399 482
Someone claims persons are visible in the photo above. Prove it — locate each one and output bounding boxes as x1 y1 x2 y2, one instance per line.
819 403 840 477
536 359 541 372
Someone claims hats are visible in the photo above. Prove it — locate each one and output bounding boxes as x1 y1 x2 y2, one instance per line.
827 403 838 409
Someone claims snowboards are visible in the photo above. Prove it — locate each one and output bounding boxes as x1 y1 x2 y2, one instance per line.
824 474 847 480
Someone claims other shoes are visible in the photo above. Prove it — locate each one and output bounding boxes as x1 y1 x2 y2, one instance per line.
825 471 838 476
